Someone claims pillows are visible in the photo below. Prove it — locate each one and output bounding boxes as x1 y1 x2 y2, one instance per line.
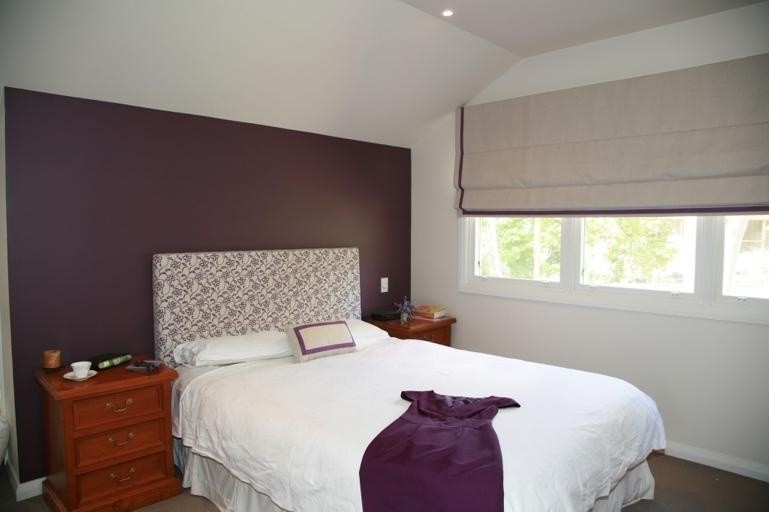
172 316 392 370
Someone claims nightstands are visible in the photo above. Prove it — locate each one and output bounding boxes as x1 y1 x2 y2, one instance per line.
33 355 182 510
367 313 456 346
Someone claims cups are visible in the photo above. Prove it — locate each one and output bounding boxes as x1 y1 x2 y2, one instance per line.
71 361 91 380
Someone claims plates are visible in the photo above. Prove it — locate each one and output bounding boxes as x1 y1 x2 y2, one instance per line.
64 368 98 382
125 359 161 374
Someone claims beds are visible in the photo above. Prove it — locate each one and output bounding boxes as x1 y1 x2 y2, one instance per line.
152 247 669 511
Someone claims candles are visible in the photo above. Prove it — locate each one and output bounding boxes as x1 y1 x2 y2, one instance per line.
42 351 61 365
